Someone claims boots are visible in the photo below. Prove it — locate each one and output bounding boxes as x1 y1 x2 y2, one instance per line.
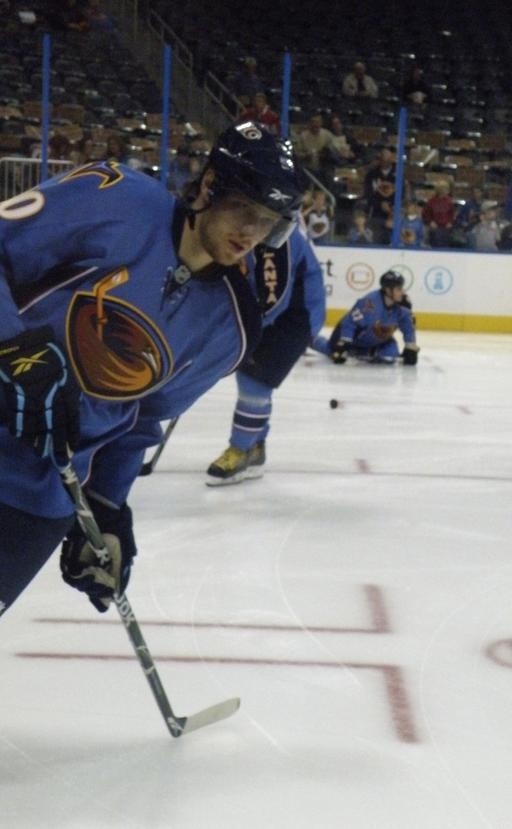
203 440 267 478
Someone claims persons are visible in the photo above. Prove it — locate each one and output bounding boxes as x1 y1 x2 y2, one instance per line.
307 269 421 364
204 225 326 486
0 117 306 614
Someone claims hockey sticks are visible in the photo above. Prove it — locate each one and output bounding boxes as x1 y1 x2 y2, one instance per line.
53 445 240 737
139 416 178 475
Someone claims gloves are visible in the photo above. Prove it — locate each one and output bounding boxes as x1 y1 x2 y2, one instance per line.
330 341 348 364
0 322 88 470
399 344 421 366
59 503 138 613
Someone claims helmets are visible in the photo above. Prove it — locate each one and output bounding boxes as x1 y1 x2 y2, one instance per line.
380 271 404 288
202 114 306 250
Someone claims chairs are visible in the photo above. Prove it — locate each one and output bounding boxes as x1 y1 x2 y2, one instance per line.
1 0 212 162
213 0 512 208
